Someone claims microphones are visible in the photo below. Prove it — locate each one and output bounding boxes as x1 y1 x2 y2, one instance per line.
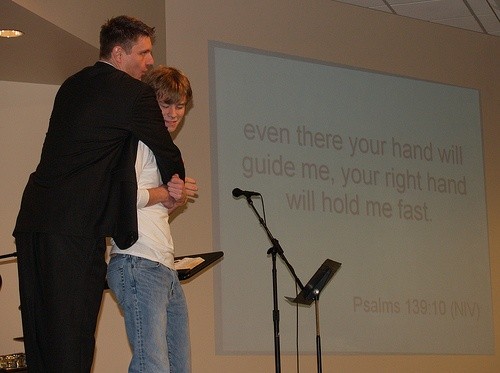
232 188 261 198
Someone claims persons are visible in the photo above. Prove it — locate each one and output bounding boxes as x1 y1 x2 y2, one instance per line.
10 14 187 373
107 66 198 373
